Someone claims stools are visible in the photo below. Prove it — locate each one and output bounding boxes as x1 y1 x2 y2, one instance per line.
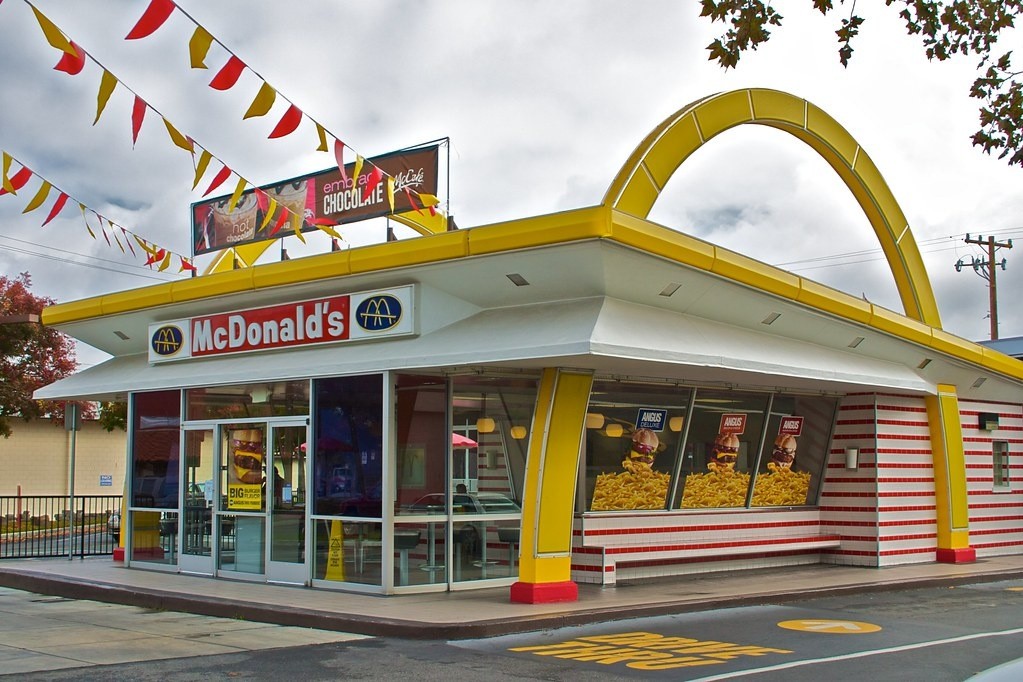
394 529 421 587
206 521 234 557
158 519 178 565
497 524 520 577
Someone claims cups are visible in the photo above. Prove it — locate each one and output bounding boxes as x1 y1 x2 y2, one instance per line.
266 187 307 235
211 192 258 247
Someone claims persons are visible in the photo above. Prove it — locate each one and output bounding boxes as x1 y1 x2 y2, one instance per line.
453 484 475 509
261 466 285 496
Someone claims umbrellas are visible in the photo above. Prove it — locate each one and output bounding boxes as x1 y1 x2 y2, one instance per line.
294 436 352 497
453 433 478 447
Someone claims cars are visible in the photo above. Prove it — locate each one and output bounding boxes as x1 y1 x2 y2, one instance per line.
394 493 521 556
107 480 235 542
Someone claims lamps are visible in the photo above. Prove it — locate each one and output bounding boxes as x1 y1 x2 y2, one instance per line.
845 445 860 472
606 404 624 438
248 383 273 404
477 393 495 433
979 412 999 430
510 424 527 439
486 450 497 470
670 409 686 432
585 384 605 430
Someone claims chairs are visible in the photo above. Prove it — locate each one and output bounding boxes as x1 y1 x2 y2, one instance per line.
324 518 382 575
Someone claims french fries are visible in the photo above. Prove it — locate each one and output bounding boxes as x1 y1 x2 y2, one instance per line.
590 468 670 509
750 468 811 507
680 469 751 507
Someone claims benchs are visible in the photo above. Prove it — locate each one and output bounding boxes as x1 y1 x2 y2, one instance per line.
568 504 841 585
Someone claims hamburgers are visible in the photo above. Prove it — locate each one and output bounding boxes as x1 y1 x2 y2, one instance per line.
231 428 265 483
625 428 659 467
770 433 797 469
710 431 740 469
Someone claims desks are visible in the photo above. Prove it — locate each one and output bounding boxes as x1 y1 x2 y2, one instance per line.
185 505 213 556
400 502 462 572
